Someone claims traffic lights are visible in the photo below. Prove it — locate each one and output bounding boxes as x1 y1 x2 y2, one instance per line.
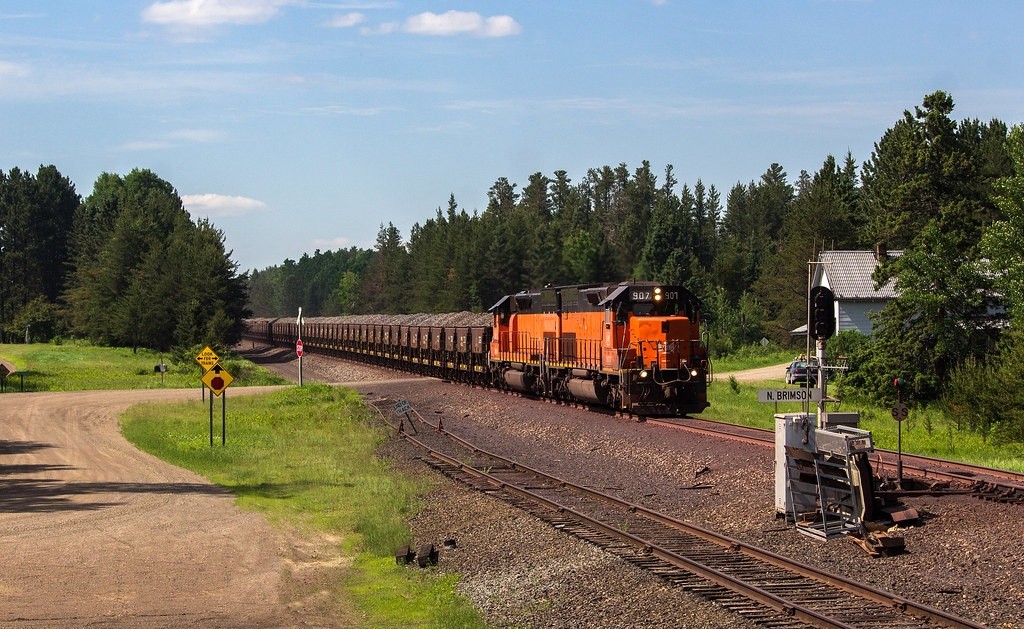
810 287 835 339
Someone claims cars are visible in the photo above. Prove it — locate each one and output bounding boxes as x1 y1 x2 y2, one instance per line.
785 361 818 384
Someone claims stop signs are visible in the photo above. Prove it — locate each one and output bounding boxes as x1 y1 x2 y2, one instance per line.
296 340 303 358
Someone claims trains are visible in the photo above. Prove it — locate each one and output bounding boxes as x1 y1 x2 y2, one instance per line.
243 278 714 418
